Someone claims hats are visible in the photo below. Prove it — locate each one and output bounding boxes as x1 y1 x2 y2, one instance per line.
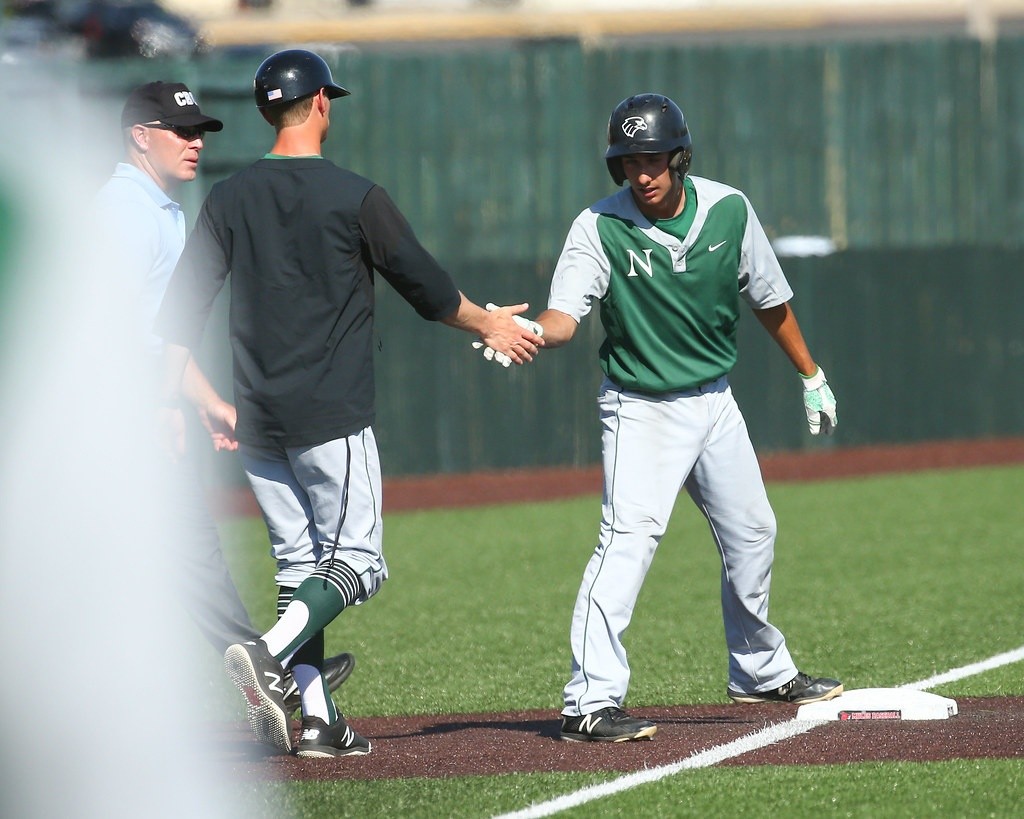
120 80 225 132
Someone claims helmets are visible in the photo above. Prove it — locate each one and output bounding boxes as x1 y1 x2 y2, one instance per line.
254 50 351 103
603 94 694 187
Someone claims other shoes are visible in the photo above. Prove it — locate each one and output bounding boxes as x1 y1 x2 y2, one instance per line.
279 652 356 718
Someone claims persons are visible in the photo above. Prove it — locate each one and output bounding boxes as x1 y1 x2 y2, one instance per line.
155 49 545 758
56 78 355 737
471 93 845 742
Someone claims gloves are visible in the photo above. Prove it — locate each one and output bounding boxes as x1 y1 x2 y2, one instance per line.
471 302 544 366
797 363 842 439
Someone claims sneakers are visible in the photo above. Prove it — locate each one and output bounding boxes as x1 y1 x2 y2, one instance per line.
224 638 294 752
724 673 845 705
559 707 657 743
295 709 376 757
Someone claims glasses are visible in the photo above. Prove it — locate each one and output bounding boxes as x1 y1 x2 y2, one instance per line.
141 123 206 138
